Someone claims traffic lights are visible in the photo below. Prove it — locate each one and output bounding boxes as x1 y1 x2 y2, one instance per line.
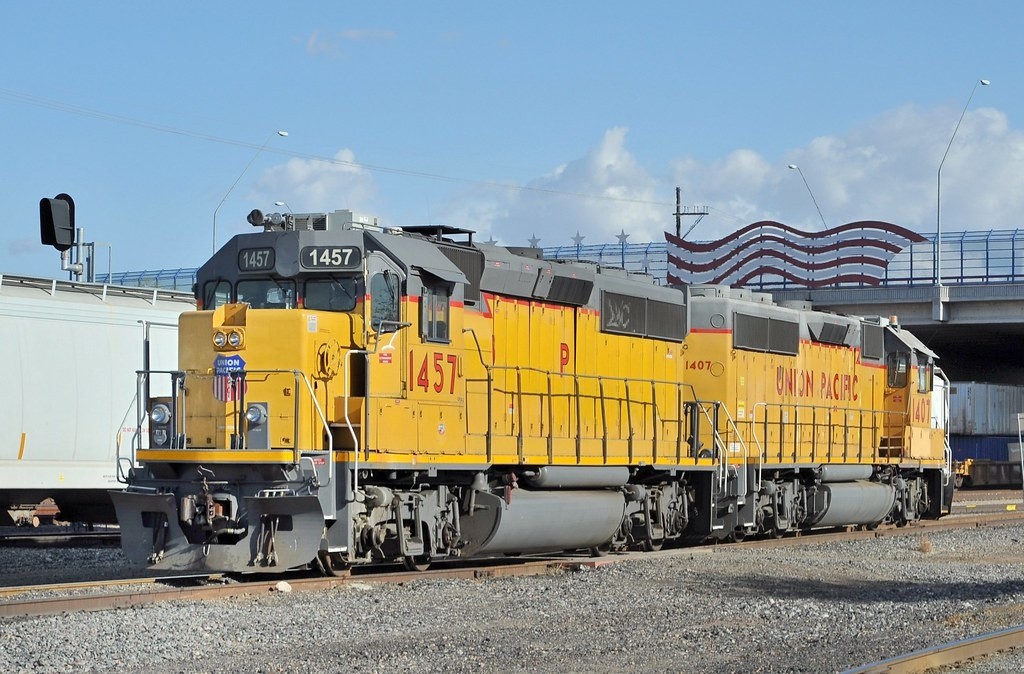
40 192 75 253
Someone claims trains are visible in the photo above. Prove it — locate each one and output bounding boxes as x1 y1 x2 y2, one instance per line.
108 206 959 581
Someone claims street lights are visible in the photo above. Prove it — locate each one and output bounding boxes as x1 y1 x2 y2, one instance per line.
212 131 288 255
936 79 991 284
788 165 852 265
